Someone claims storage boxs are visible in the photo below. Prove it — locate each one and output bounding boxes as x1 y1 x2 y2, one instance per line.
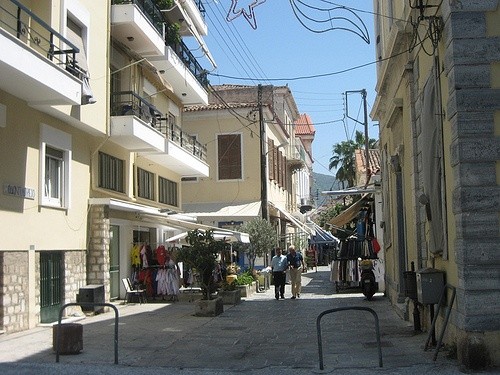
360 260 372 271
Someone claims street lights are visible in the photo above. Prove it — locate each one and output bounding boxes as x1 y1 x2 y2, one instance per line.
259 140 291 289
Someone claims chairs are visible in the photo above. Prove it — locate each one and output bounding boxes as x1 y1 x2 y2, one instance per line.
122 277 148 305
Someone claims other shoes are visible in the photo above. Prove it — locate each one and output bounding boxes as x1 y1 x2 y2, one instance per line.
291 296 296 299
281 295 284 299
297 294 300 298
275 294 279 300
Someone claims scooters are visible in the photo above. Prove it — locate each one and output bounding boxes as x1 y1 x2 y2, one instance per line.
357 257 380 300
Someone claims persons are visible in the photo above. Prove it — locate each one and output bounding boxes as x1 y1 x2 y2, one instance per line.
287 245 305 299
271 248 289 300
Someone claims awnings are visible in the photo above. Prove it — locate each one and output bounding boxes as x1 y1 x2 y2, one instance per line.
145 189 374 244
